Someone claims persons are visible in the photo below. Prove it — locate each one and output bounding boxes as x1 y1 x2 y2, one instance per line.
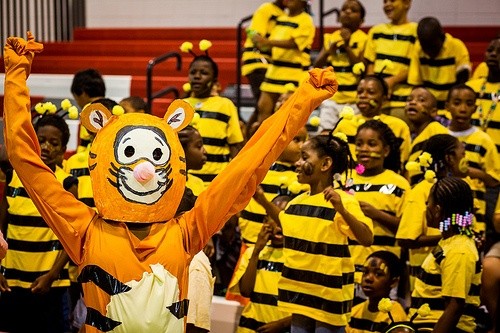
4 32 339 333
0 1 500 333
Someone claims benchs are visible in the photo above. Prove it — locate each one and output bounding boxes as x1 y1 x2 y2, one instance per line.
0 24 500 199
